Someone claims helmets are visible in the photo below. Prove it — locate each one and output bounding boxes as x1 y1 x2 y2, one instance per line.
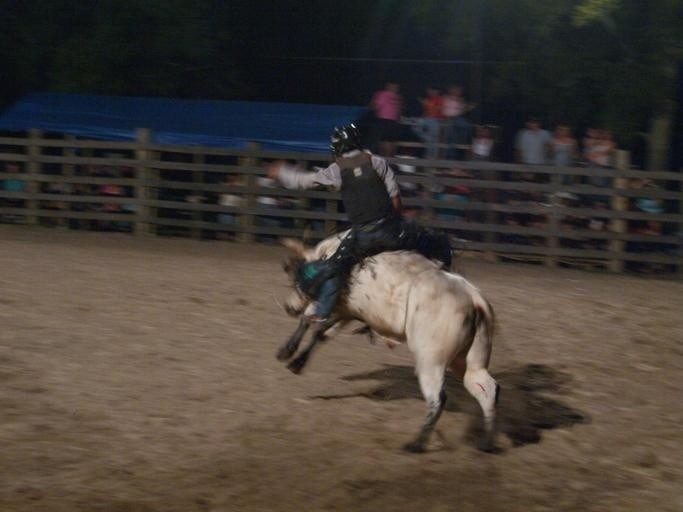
329 124 361 154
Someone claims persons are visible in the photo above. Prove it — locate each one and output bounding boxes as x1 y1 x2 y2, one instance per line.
267 123 450 323
213 172 311 244
46 165 124 229
367 78 663 253
1 161 26 206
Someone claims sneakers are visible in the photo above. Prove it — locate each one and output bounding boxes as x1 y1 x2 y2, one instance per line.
307 315 327 322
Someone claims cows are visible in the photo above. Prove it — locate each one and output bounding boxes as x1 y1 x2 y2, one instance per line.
275 227 500 454
